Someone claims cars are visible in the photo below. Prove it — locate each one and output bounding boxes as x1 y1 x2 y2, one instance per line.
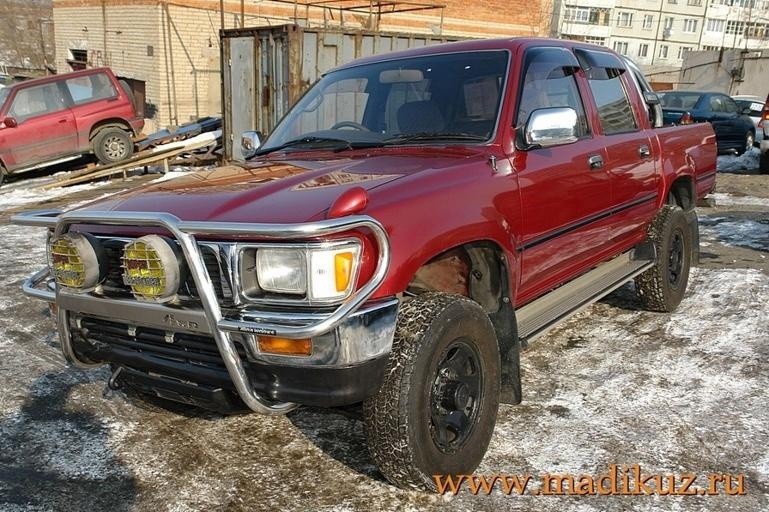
656 90 769 173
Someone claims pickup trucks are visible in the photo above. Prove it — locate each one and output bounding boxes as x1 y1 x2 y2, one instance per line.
22 37 717 495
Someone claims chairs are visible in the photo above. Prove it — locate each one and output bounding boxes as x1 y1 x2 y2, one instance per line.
395 99 446 138
670 97 683 108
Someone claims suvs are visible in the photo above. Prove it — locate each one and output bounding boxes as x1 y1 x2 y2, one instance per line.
1 67 145 187
